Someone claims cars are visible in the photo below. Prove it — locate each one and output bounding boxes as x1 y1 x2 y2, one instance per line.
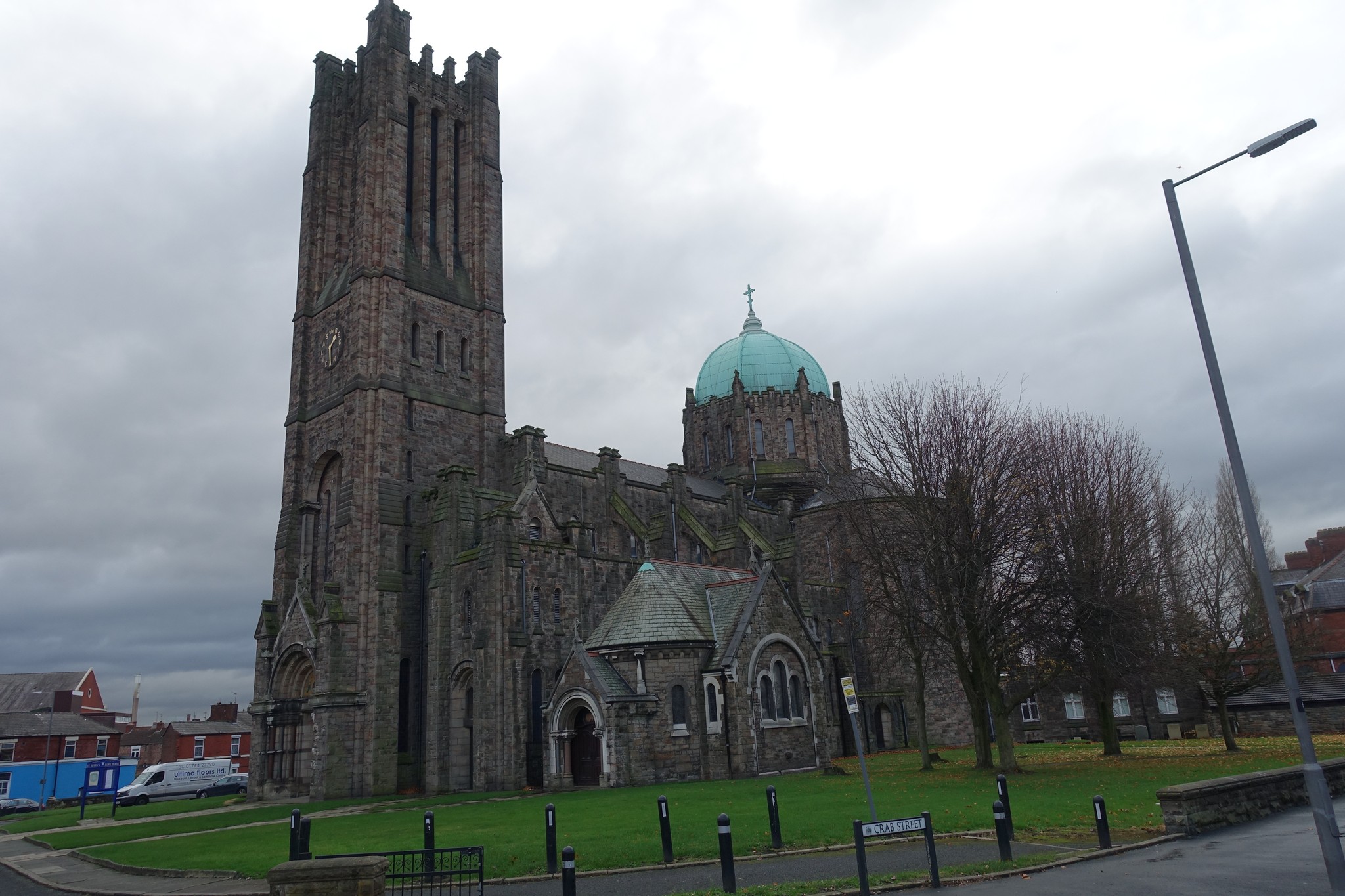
197 772 249 798
0 798 44 816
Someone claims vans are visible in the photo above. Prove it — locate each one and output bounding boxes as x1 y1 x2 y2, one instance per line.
116 759 233 807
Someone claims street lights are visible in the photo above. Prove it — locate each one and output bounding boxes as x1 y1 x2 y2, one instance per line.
1161 118 1344 896
